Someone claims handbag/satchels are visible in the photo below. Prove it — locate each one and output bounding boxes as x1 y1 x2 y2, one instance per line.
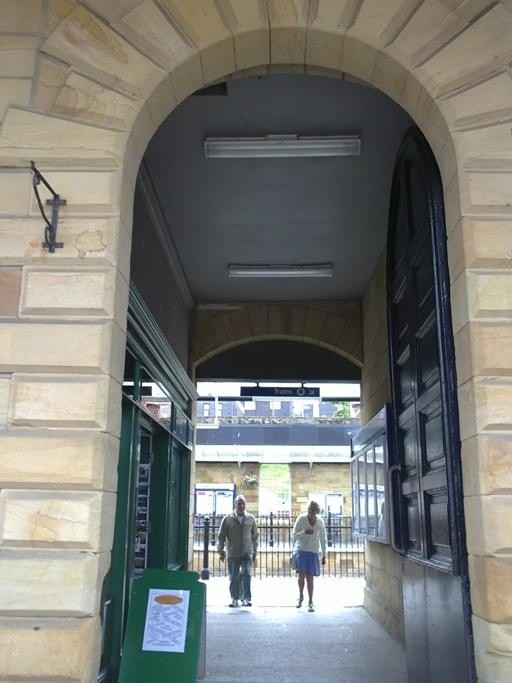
289 557 296 570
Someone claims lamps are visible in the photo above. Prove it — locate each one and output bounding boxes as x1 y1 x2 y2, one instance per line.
228 264 333 278
203 131 362 158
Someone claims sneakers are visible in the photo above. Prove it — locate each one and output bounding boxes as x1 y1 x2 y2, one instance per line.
308 604 315 612
296 599 303 608
229 600 238 607
242 601 252 606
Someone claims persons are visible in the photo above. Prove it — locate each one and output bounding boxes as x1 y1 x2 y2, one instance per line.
217 495 258 606
291 501 328 612
